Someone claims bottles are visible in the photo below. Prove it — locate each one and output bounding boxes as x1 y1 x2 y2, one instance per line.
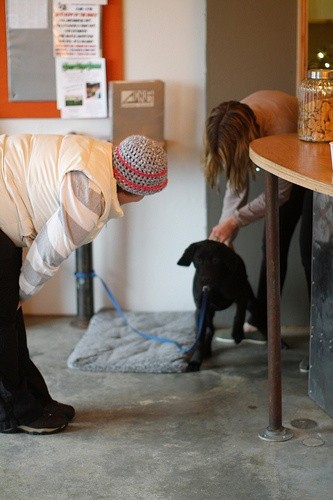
297 69 333 143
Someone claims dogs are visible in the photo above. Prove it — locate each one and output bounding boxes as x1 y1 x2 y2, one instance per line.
171 238 267 374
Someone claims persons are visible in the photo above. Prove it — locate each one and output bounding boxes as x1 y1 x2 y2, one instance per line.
198 89 313 376
1 130 173 435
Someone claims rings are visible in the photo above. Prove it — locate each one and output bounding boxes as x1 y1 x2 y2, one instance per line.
213 236 219 240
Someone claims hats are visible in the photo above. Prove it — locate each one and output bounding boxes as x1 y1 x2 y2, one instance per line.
111 135 168 195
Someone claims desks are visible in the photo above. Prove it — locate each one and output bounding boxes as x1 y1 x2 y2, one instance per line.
249 130 333 442
